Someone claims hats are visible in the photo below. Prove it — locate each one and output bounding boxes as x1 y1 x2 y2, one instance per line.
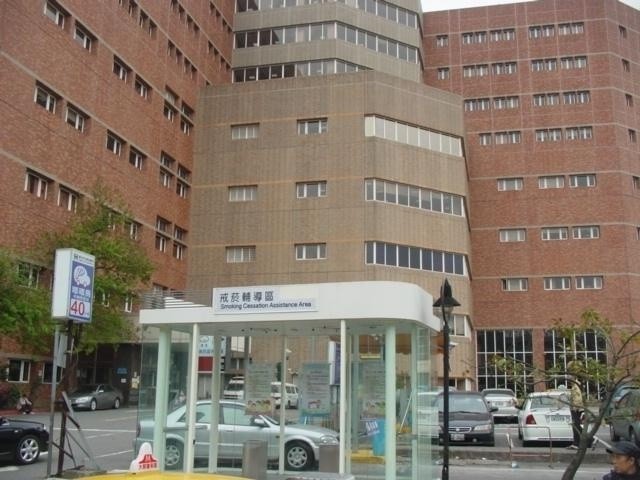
605 441 640 459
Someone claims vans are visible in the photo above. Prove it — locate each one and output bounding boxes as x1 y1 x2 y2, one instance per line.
271 382 300 409
224 375 245 401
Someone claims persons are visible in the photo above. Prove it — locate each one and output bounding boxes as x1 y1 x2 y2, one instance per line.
602 441 640 479
16 394 33 415
566 375 587 450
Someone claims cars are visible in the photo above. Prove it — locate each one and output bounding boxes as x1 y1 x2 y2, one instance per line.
480 387 520 419
70 440 259 480
600 384 640 423
430 389 500 447
608 390 640 445
132 398 339 472
0 414 50 467
62 382 125 412
514 389 594 446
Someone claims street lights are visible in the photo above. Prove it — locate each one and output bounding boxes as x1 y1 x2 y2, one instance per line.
431 277 462 480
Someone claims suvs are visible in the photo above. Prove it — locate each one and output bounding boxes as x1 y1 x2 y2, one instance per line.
416 384 458 440
